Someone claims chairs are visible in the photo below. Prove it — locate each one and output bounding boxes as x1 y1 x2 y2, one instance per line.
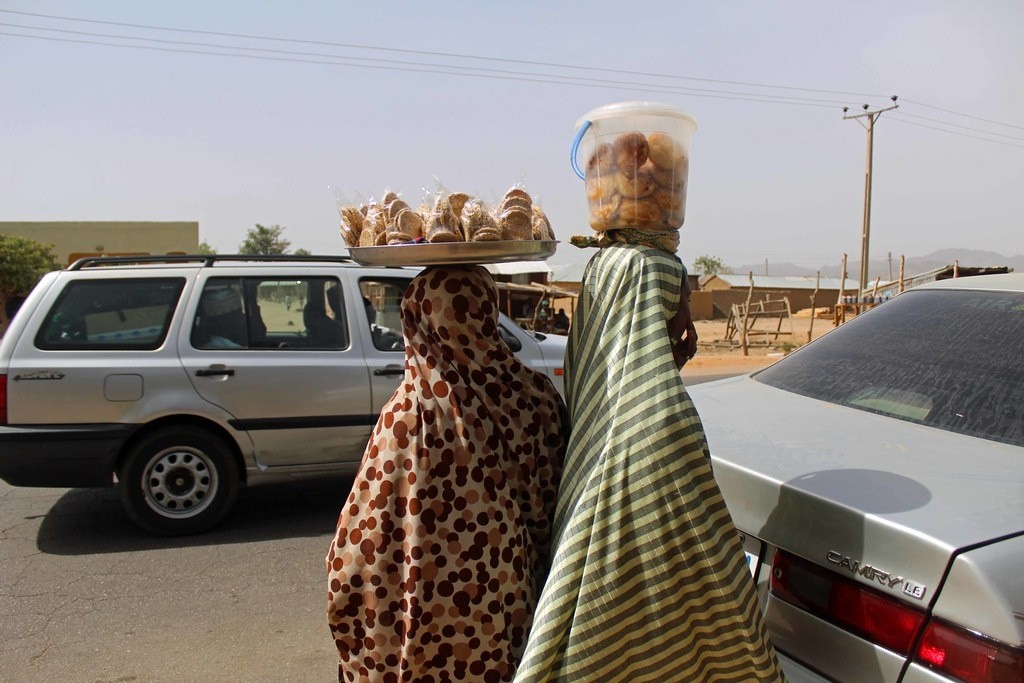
303 301 346 350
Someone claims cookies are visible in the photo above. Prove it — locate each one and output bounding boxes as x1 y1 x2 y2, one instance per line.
336 189 557 248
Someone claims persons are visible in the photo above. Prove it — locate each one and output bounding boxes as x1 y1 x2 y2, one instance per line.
4 289 24 320
523 297 569 336
326 264 574 683
198 288 249 350
509 244 789 683
363 296 395 350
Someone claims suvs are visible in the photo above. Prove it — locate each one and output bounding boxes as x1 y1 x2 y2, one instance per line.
0 252 570 538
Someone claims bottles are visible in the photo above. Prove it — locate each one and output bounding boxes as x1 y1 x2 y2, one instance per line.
841 294 889 304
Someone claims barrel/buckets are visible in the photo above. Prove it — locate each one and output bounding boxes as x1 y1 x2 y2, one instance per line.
568 100 699 230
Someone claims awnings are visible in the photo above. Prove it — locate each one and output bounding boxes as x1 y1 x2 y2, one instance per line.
479 261 553 274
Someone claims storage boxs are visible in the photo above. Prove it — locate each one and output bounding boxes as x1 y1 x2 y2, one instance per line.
84 301 171 348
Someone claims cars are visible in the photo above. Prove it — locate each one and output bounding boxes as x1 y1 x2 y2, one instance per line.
687 273 1024 683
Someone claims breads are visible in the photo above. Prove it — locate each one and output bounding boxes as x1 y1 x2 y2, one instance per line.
587 131 689 230
